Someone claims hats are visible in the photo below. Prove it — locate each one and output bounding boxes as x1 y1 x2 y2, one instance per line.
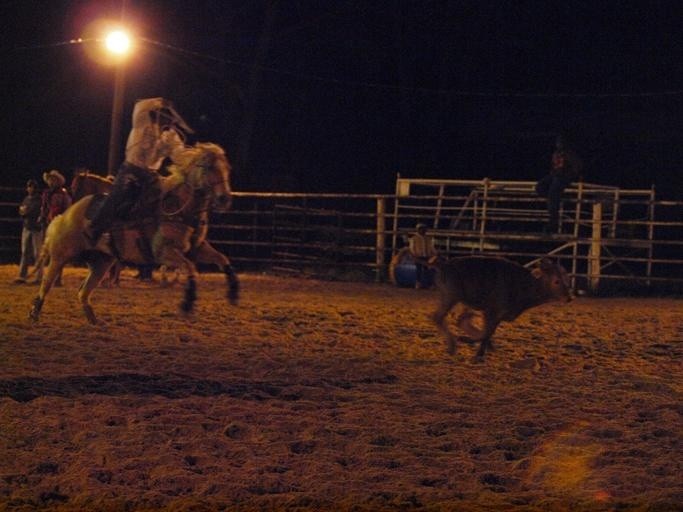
40 169 65 186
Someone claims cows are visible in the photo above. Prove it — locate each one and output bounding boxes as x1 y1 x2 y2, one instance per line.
429 249 575 365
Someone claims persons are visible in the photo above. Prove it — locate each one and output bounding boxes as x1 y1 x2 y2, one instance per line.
82 95 189 247
408 223 437 290
12 179 42 284
35 170 72 286
535 134 578 236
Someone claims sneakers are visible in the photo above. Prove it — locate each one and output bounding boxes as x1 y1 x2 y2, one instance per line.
81 227 96 241
13 279 61 287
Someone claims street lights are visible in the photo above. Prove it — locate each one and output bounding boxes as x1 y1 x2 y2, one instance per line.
103 30 130 175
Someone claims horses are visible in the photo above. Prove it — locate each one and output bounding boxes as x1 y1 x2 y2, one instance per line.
21 140 240 326
